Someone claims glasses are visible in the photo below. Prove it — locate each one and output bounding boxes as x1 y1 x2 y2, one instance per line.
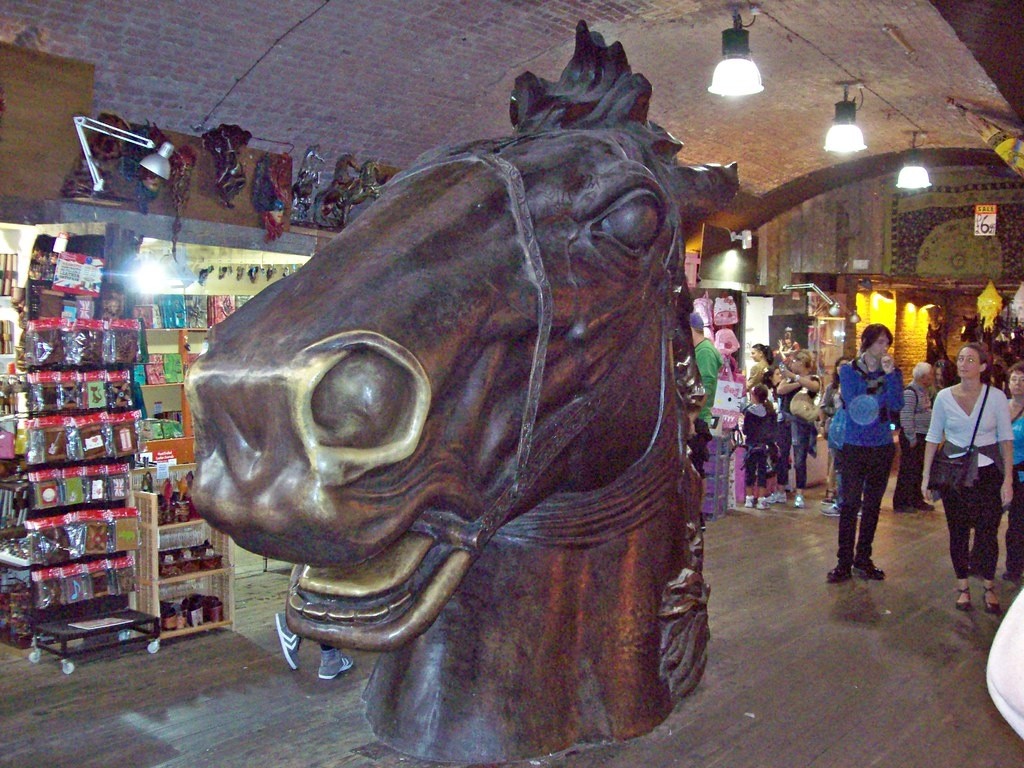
934 361 945 368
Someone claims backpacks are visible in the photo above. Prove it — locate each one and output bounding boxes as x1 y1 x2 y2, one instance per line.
891 384 919 424
692 290 740 354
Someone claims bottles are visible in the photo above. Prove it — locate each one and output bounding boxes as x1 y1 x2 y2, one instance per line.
778 360 792 383
140 471 223 631
963 445 980 489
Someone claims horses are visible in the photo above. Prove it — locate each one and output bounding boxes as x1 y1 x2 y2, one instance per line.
186 13 741 760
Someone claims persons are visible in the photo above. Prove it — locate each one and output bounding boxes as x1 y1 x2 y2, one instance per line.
275 610 354 679
746 339 803 477
687 312 724 530
921 342 1013 614
826 324 904 582
987 589 1024 742
927 333 1020 410
1002 361 1024 583
819 356 865 518
767 349 822 507
893 362 935 514
742 383 777 510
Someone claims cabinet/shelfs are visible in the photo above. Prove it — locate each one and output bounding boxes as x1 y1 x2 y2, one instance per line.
0 197 336 665
702 434 732 520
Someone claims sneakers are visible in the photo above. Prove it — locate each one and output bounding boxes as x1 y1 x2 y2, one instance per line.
820 503 840 516
317 649 353 679
745 495 755 507
766 488 786 503
757 497 770 509
275 612 301 670
794 494 804 508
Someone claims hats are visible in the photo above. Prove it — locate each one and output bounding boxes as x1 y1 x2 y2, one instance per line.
690 312 704 330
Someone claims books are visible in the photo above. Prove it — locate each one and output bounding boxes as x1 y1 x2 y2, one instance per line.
134 353 200 385
153 411 182 426
153 295 254 330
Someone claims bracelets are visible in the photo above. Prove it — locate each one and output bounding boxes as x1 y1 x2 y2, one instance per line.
794 374 801 383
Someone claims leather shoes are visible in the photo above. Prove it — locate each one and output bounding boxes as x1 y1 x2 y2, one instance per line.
826 566 852 580
853 560 885 580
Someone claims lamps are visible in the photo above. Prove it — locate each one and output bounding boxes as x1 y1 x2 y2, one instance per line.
925 301 936 309
824 84 868 154
708 3 765 96
895 130 932 189
73 115 174 192
730 229 753 250
876 290 894 300
783 283 840 317
811 294 861 324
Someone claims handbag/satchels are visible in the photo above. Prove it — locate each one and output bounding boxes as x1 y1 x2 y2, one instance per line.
789 375 824 422
928 447 972 498
712 355 746 428
827 406 847 450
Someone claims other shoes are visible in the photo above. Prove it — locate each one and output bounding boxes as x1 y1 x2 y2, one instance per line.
956 586 971 611
821 498 836 504
982 586 1000 613
915 500 934 510
893 504 918 513
1002 569 1022 579
